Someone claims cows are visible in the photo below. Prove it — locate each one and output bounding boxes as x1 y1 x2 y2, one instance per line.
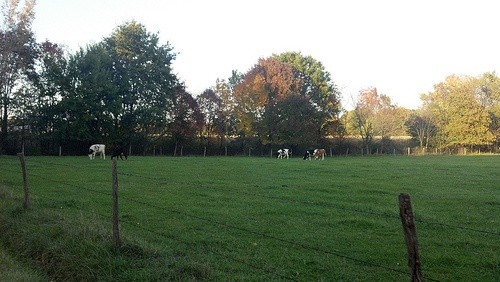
110 143 128 160
88 143 106 161
302 149 316 160
273 148 292 159
313 148 327 160
